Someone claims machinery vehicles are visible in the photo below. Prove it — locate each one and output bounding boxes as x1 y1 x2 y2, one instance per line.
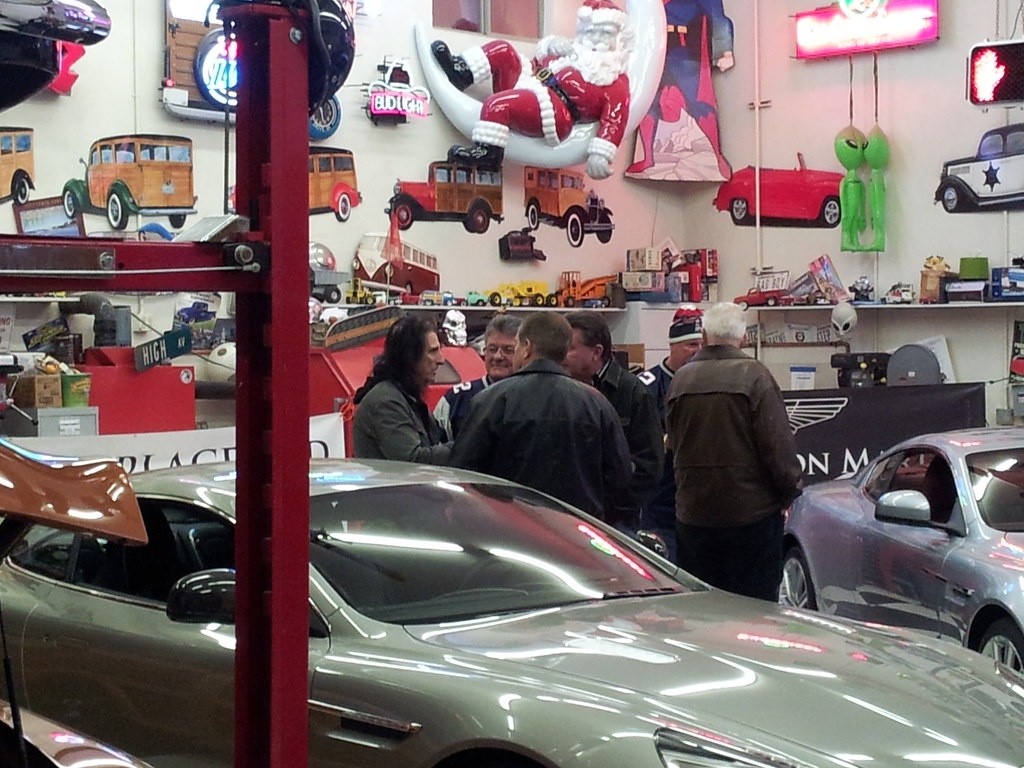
557 270 618 309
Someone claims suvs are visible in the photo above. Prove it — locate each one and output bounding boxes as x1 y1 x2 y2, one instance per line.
934 122 1024 214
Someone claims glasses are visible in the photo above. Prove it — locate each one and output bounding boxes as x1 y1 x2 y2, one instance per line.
485 343 514 356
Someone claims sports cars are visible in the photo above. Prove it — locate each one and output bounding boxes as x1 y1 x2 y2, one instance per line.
712 152 846 229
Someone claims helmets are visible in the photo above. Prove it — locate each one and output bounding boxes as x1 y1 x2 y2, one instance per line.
210 0 355 118
207 343 237 382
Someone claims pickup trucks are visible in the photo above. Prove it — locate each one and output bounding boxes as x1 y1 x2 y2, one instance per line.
733 287 791 311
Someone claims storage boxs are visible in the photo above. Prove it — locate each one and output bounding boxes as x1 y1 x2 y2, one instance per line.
6 373 62 410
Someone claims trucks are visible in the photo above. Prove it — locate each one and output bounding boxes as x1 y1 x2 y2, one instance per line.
345 276 549 308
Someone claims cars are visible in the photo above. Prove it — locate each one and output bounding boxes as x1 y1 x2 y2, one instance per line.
1 126 36 208
0 457 1024 768
226 146 362 227
0 0 112 47
385 157 505 234
60 133 198 231
778 426 1024 678
523 165 615 248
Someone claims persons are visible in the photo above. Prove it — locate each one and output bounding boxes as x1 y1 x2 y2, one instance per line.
663 302 803 600
431 0 635 180
341 314 454 606
433 305 703 613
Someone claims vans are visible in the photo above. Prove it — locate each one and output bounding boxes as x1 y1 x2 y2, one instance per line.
886 287 912 305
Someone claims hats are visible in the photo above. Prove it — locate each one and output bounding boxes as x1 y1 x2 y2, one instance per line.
669 316 703 344
